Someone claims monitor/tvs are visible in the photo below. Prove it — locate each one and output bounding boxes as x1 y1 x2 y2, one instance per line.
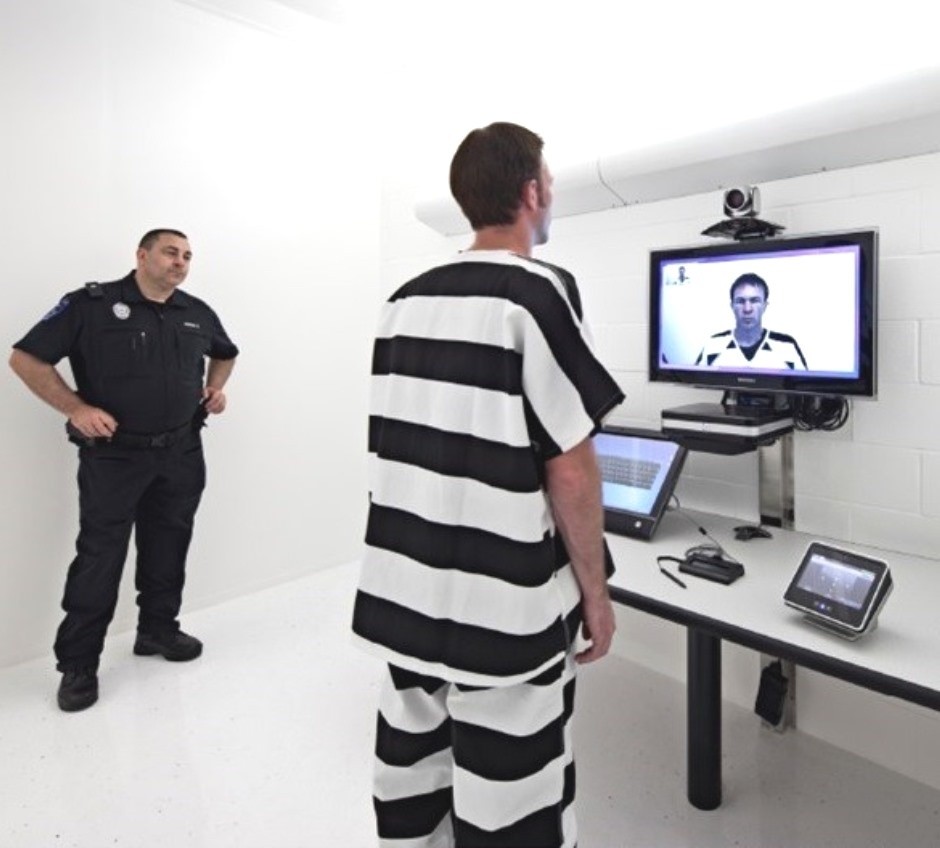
591 424 689 540
647 229 881 400
783 541 889 631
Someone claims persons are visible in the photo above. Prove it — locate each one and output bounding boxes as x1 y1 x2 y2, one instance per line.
8 228 239 712
352 122 626 848
694 273 809 372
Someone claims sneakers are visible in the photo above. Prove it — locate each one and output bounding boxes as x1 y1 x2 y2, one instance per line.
57 661 98 711
134 630 203 662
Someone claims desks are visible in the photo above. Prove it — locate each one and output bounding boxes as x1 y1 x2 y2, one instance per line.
604 508 939 809
